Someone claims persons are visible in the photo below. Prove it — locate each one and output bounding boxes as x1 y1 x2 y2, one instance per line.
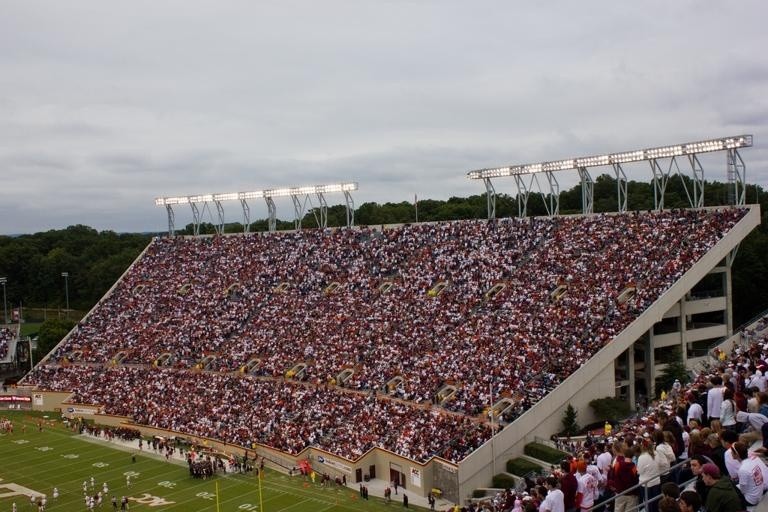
1 416 13 433
454 316 768 512
187 455 266 480
0 327 16 375
289 461 369 501
18 205 750 462
385 480 435 510
11 476 129 511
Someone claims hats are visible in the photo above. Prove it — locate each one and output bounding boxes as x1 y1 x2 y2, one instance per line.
702 463 718 473
576 459 587 470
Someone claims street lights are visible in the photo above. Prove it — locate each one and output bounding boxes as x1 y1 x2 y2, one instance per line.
0 276 9 324
61 271 70 318
